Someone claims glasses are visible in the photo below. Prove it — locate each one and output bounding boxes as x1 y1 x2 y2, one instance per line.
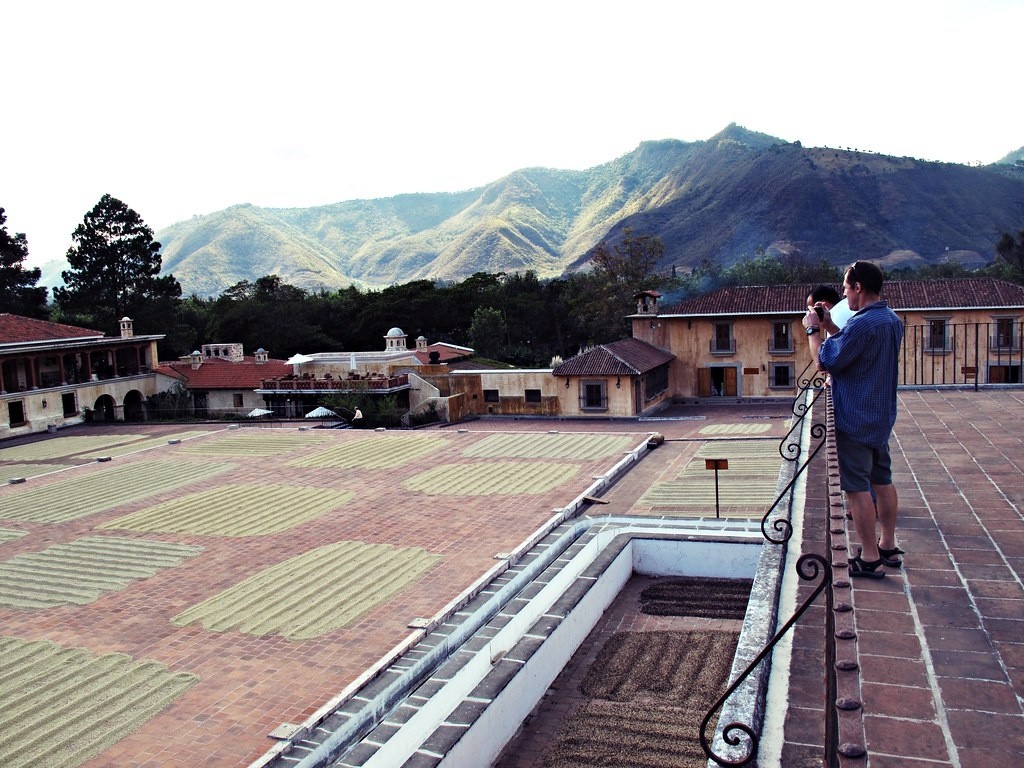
852 260 862 284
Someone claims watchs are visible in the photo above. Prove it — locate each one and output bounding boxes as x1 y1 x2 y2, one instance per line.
806 327 821 334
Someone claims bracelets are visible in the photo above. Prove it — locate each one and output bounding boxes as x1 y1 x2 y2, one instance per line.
805 325 819 330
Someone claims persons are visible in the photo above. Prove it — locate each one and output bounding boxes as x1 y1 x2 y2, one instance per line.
800 261 906 581
351 407 363 429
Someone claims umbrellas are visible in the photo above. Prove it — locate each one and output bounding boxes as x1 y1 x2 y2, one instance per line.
349 354 357 370
284 353 313 376
247 408 274 423
305 407 338 426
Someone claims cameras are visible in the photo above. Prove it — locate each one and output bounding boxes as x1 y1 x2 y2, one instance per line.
812 306 824 323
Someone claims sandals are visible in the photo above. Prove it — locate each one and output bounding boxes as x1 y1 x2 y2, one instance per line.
848 554 886 580
857 537 906 566
